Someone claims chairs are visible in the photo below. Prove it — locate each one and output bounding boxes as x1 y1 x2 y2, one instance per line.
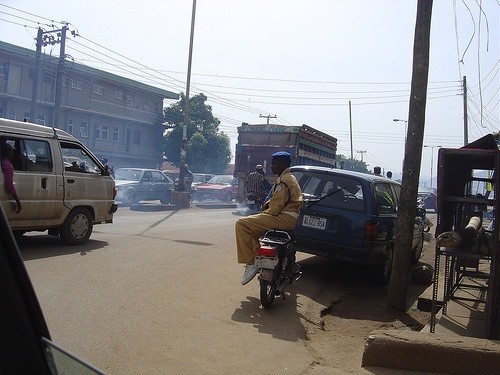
327 188 344 197
31 154 48 171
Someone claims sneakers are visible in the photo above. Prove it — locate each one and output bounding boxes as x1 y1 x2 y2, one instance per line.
241 264 257 285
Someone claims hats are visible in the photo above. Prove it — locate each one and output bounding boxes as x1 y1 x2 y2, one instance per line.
272 151 291 157
256 165 263 169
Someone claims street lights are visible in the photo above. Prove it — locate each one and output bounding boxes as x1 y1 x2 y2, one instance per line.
393 118 407 146
424 144 442 187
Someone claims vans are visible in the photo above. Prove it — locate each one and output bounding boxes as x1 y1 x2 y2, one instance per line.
259 165 430 286
0 116 119 246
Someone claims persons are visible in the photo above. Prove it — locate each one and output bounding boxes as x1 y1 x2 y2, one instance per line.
235 151 304 285
0 143 22 214
183 163 193 191
246 164 272 195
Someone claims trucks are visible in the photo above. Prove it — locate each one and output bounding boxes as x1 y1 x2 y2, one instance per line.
233 121 338 205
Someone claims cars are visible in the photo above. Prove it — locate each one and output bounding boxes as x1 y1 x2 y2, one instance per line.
162 169 239 202
417 191 437 212
113 167 175 207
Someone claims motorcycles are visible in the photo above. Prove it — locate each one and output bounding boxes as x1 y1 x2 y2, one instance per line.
253 197 321 309
243 192 269 215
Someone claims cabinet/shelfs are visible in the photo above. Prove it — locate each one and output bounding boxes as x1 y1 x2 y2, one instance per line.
430 148 500 339
